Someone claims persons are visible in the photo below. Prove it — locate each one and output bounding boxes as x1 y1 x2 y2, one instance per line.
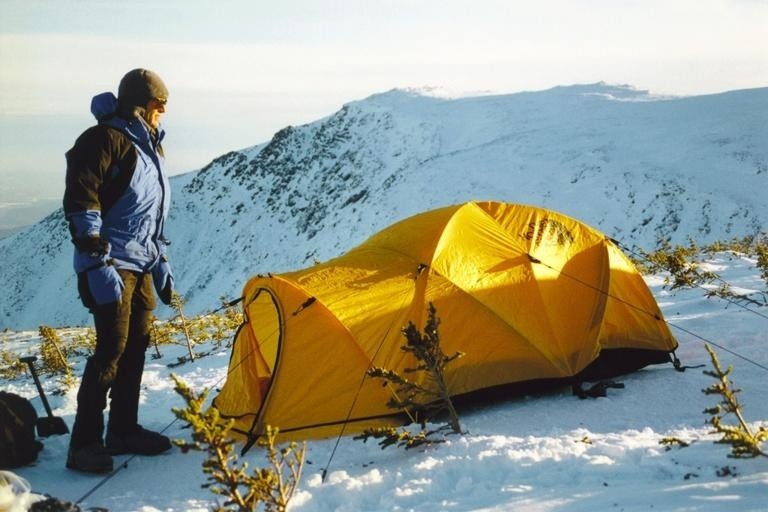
63 68 176 476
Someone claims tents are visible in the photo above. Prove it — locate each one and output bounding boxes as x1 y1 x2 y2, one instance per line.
207 199 680 458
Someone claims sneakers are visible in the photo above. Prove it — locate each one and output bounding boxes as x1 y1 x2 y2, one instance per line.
104 425 172 456
62 443 116 475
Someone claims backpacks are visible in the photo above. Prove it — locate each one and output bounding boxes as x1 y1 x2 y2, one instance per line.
0 391 42 468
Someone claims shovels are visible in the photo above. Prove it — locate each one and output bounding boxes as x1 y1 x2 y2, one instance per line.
19 357 68 436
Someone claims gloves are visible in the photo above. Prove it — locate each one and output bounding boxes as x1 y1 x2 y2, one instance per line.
151 240 175 306
72 235 125 309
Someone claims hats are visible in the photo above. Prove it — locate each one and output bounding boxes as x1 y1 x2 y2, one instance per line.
118 68 168 113
